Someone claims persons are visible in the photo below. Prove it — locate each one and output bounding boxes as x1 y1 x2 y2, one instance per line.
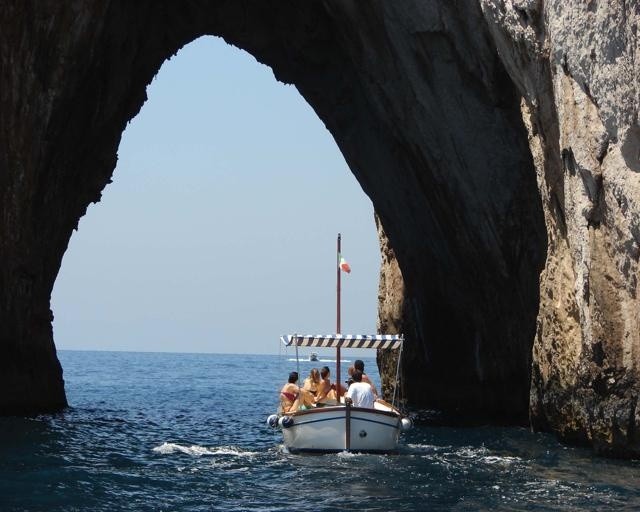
315 365 346 404
348 359 378 403
304 367 321 397
344 371 374 409
280 370 315 414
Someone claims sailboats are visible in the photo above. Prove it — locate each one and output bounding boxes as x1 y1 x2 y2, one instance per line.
277 233 405 456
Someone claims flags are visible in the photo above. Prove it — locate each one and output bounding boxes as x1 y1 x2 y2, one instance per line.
337 252 351 275
